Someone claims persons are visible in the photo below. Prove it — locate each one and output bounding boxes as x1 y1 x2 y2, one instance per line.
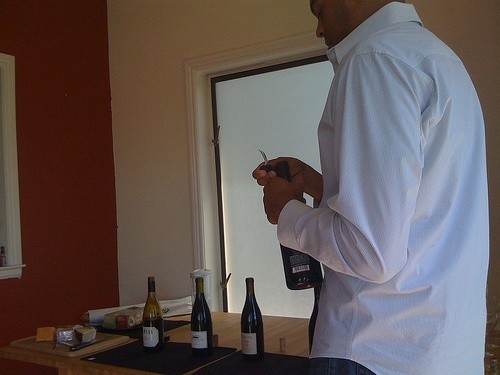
253 0 490 375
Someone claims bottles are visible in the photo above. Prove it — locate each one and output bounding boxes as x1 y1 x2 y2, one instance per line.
142 277 164 352
275 161 313 290
241 278 264 360
191 277 214 357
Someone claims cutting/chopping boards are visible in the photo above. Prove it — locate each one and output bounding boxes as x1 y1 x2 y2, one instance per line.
10 332 129 357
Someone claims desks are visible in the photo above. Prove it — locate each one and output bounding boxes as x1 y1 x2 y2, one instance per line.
0 311 310 375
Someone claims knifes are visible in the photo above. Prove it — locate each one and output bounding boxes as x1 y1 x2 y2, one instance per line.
71 338 104 350
